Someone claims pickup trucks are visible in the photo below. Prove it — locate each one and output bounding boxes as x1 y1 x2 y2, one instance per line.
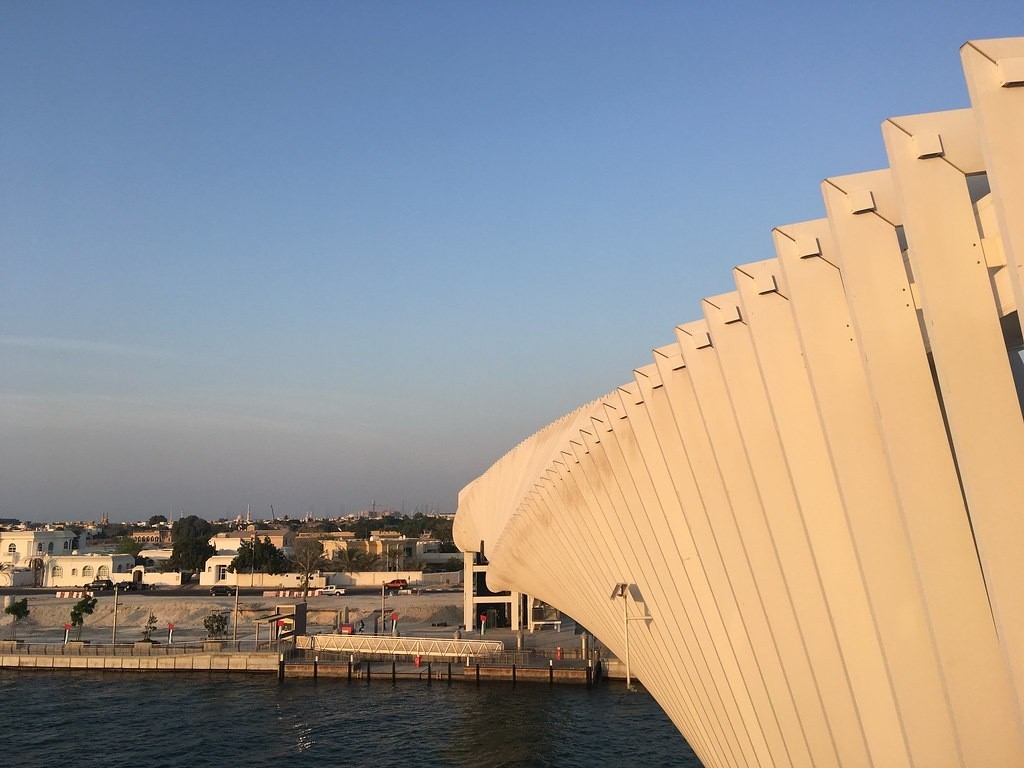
315 585 347 596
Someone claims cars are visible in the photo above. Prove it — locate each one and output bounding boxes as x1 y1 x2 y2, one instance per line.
113 581 137 592
385 579 408 590
209 585 235 597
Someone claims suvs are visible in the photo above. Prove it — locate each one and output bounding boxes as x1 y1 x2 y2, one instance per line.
84 580 113 591
